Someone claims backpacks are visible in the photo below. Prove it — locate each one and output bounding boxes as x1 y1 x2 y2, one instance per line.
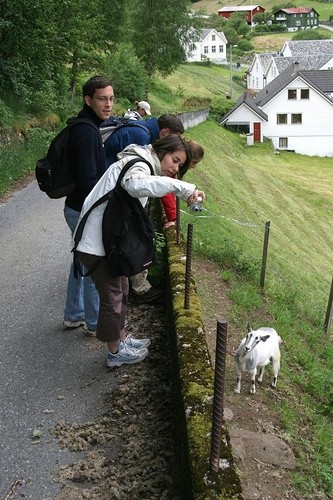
35 118 102 199
99 117 150 145
70 158 158 279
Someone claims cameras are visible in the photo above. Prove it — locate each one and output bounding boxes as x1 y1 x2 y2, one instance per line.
190 197 202 212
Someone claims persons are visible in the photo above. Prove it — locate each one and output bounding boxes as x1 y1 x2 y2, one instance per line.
62 76 129 337
99 99 204 234
71 133 206 368
225 90 231 99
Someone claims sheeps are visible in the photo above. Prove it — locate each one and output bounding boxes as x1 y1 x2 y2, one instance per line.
232 323 283 394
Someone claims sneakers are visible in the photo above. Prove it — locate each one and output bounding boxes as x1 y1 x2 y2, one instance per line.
107 334 151 367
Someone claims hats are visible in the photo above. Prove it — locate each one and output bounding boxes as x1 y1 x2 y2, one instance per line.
138 101 151 115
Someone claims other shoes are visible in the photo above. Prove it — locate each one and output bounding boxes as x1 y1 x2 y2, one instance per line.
127 286 167 305
86 318 128 336
62 319 86 328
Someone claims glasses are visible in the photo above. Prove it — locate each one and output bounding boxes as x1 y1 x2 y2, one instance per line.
92 96 114 102
